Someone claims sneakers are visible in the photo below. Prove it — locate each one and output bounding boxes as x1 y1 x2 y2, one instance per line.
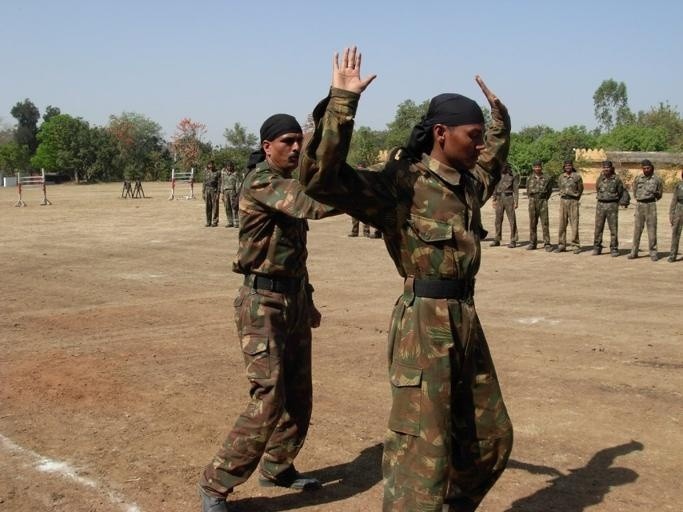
627 253 639 260
650 254 659 261
544 244 554 252
205 222 240 228
508 241 517 248
592 248 602 255
526 243 537 250
610 247 620 257
572 247 581 254
668 258 676 262
490 240 500 247
348 229 382 239
555 247 566 253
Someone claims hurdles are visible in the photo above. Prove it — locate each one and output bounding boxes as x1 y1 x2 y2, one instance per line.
13 168 51 206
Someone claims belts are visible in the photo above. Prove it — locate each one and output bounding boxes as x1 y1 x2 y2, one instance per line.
243 273 301 295
412 278 476 301
530 194 547 199
560 195 578 200
598 199 618 203
637 197 656 203
495 192 513 196
678 198 683 203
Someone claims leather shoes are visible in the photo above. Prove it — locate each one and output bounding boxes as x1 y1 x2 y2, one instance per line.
198 483 227 512
258 473 323 491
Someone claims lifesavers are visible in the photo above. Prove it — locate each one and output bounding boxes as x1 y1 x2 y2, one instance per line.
168 168 195 200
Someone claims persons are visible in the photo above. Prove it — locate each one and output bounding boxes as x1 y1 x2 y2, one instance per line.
374 228 382 238
554 160 582 255
628 160 660 262
348 162 371 237
620 187 631 209
668 171 683 262
591 160 622 257
198 113 386 512
299 45 514 512
201 159 221 227
221 161 240 227
489 161 520 248
527 160 553 252
